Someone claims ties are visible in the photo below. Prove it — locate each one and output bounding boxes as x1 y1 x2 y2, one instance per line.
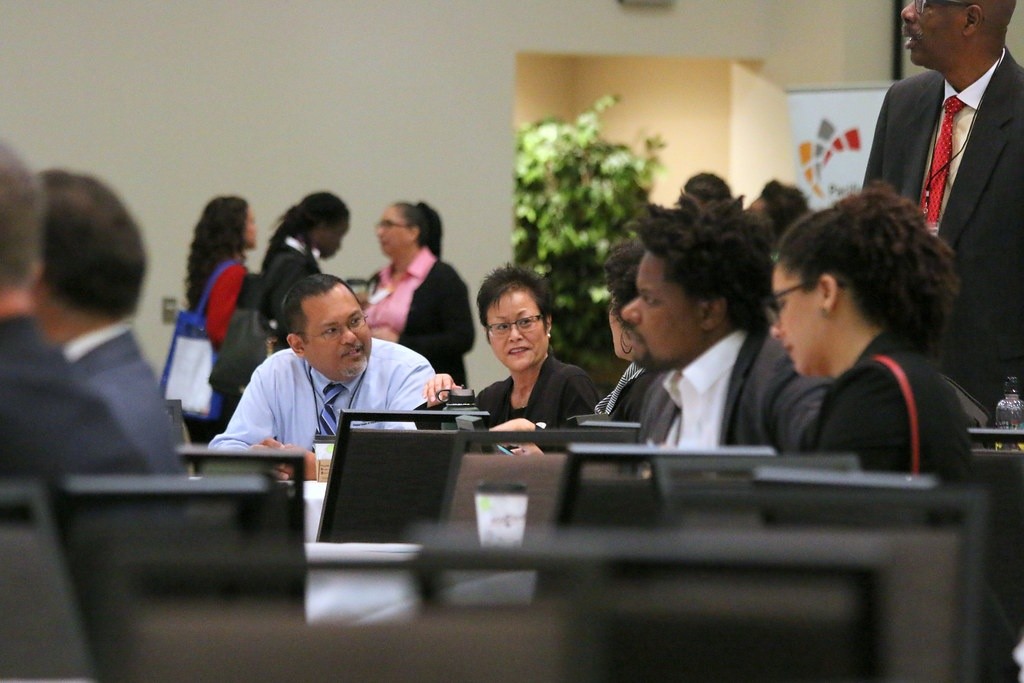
312 383 346 452
920 95 967 233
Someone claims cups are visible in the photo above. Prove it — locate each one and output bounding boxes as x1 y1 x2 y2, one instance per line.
347 279 367 306
475 481 528 545
436 388 481 431
312 434 336 481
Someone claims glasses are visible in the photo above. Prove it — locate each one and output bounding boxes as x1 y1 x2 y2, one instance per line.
379 220 409 231
766 278 845 321
487 312 543 337
915 0 986 22
296 313 370 342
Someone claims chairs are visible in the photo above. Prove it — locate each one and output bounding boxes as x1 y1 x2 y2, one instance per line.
0 384 1024 683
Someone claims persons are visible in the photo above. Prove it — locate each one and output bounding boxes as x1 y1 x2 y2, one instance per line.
0 139 149 519
362 201 477 389
208 274 436 482
862 0 1024 451
414 261 600 494
34 168 187 478
258 191 371 354
183 195 257 476
601 173 974 477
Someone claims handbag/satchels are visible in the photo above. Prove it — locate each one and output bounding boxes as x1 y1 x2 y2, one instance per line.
208 249 305 398
161 262 241 421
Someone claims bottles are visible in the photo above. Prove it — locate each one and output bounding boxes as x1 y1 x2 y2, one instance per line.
995 376 1024 451
